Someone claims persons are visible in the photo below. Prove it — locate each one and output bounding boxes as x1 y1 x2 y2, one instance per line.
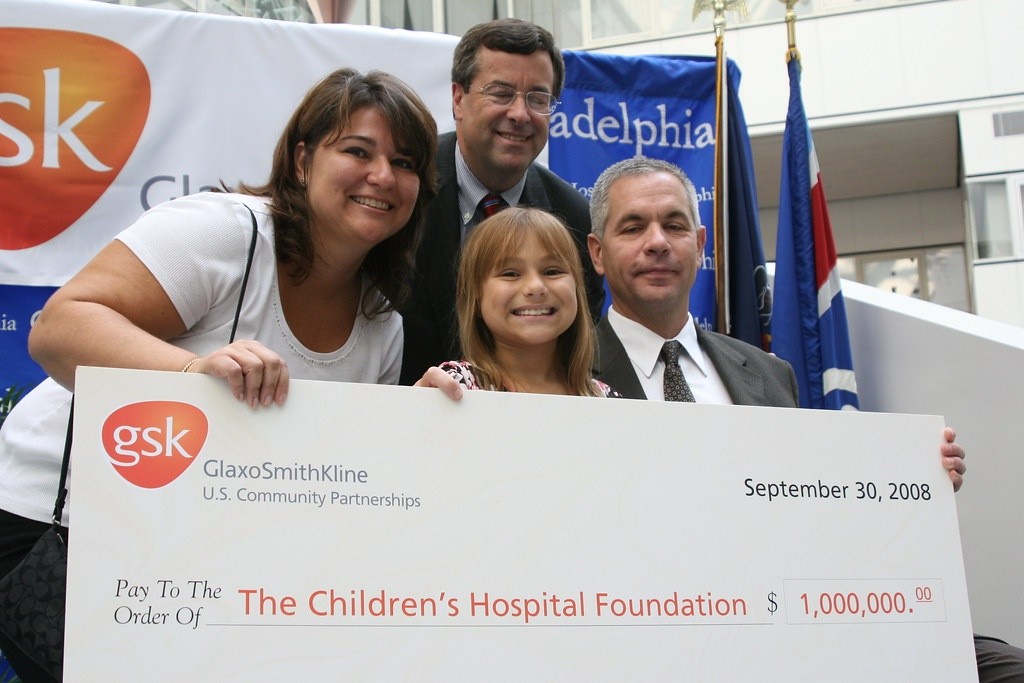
395 18 606 386
0 70 439 683
584 154 1023 683
413 206 623 399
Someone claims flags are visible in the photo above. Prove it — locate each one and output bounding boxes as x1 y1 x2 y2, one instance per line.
722 61 772 353
761 50 857 411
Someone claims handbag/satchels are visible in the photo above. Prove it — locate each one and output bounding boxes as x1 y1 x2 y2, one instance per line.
0 522 68 683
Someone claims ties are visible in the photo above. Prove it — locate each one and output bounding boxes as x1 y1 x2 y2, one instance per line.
661 340 695 402
479 195 502 218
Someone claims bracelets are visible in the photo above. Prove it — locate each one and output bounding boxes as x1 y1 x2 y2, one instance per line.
181 356 199 372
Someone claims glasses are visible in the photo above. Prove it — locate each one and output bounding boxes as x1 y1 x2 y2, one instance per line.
484 83 562 116
